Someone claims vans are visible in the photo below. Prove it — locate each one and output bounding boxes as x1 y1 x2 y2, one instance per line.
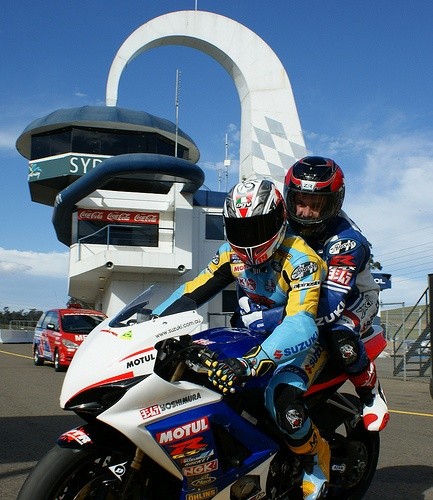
32 309 108 372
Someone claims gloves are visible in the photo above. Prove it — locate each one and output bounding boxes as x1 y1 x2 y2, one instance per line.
207 344 277 396
235 284 284 335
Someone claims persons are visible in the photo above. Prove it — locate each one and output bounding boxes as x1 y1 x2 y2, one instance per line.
127 179 330 500
230 156 389 431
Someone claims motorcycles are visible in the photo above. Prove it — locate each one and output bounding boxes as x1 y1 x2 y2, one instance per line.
15 283 388 500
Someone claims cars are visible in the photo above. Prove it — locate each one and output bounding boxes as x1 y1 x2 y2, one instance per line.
420 334 431 348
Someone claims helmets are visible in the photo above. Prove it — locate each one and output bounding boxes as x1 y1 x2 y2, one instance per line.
282 155 346 236
220 178 288 269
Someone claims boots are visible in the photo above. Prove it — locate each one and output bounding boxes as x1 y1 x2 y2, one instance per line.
347 360 390 433
284 419 332 500
